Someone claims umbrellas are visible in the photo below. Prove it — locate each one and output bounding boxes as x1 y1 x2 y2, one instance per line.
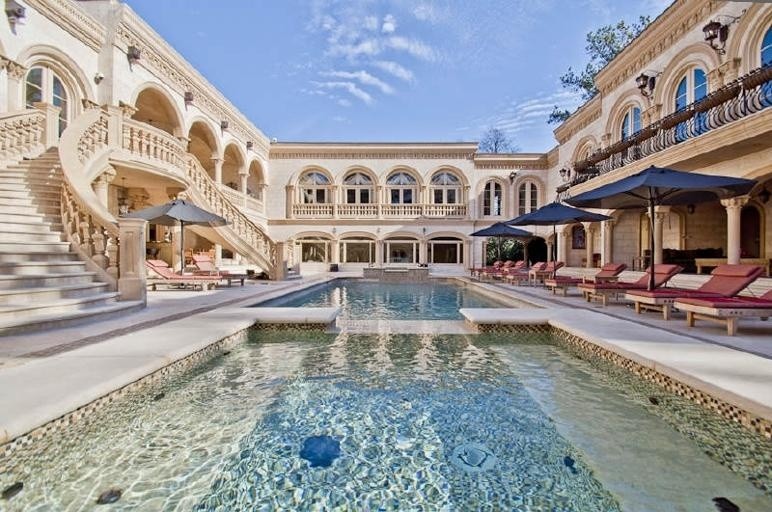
563 164 759 291
122 198 228 275
504 200 613 279
468 221 529 269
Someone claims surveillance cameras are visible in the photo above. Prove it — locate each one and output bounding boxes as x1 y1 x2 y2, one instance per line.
98 72 105 80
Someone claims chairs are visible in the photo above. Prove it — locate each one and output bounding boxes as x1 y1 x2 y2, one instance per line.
673 288 772 336
544 263 628 296
192 255 249 287
467 261 565 287
624 264 765 321
146 259 223 291
578 264 684 306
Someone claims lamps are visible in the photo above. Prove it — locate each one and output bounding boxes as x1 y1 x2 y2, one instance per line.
635 73 656 97
559 168 570 181
758 187 771 204
703 20 729 55
687 203 696 214
